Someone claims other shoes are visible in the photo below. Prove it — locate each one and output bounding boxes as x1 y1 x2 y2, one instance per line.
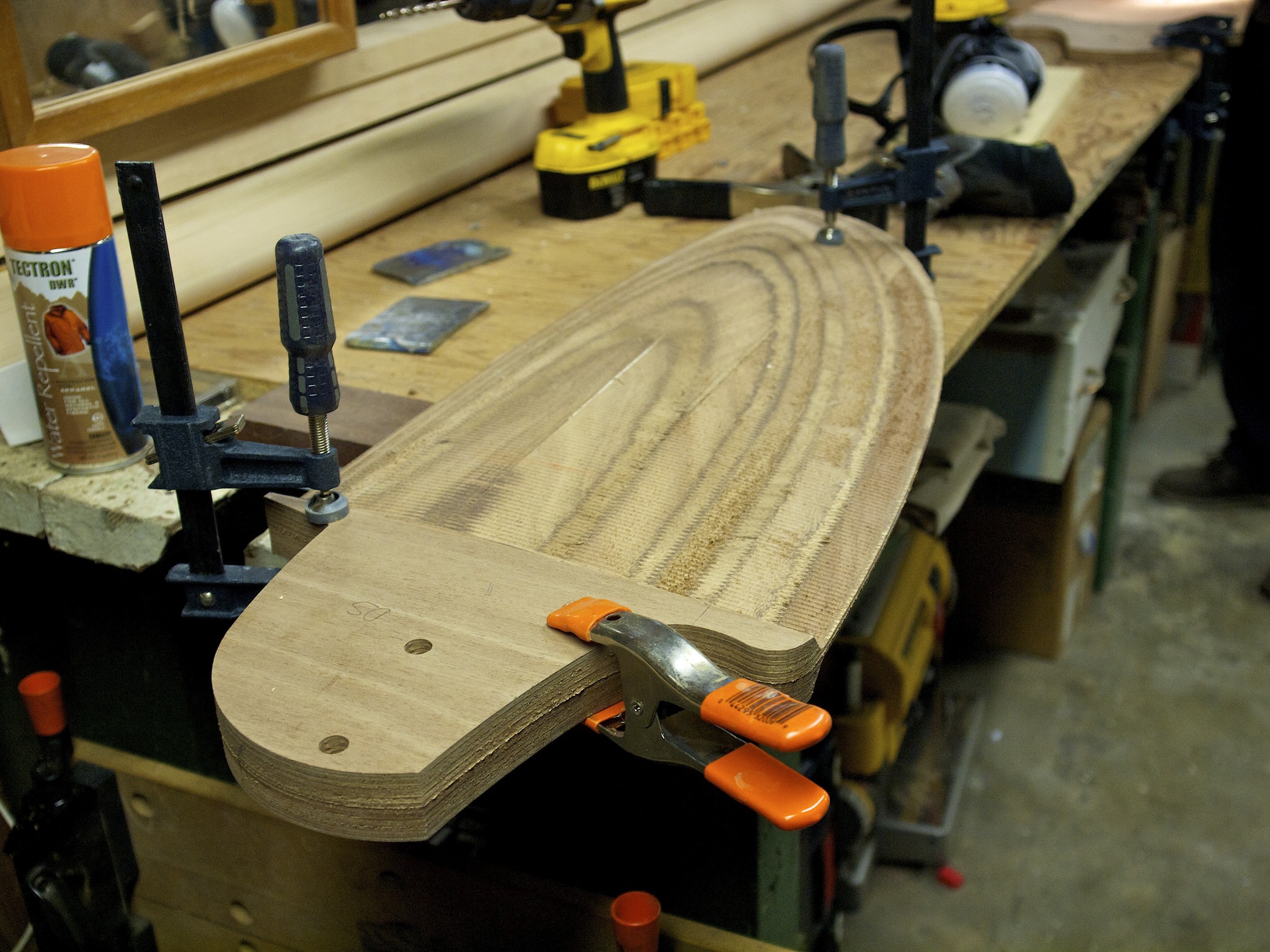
1155 459 1270 511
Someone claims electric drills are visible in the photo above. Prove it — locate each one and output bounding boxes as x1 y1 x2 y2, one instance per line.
379 0 659 225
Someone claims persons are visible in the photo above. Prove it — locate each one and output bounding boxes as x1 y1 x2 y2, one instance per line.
1153 0 1270 601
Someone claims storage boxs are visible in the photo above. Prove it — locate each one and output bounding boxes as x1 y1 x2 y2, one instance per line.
942 238 1149 660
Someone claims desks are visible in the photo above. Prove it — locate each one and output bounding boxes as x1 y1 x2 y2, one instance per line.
0 0 1205 952
